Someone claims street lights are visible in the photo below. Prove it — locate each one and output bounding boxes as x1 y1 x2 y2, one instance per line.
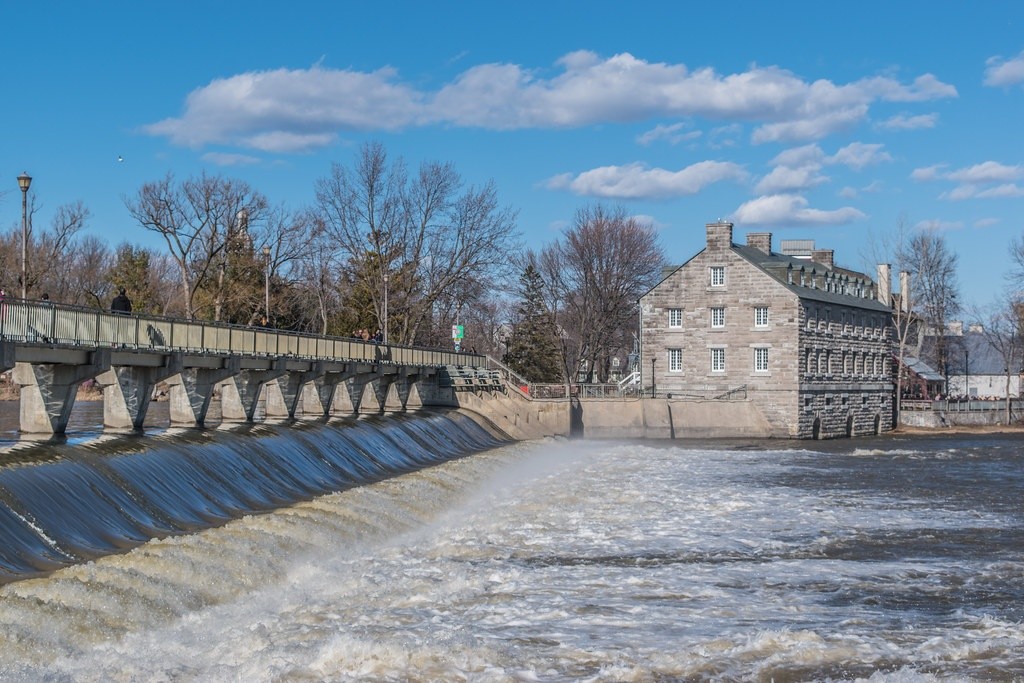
965 349 969 396
261 242 272 325
17 171 33 343
456 304 460 326
504 332 511 379
651 356 657 399
383 274 390 345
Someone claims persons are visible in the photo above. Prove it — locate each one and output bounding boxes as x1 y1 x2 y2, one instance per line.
258 314 274 330
350 328 383 343
894 391 959 403
469 346 477 354
111 287 132 316
